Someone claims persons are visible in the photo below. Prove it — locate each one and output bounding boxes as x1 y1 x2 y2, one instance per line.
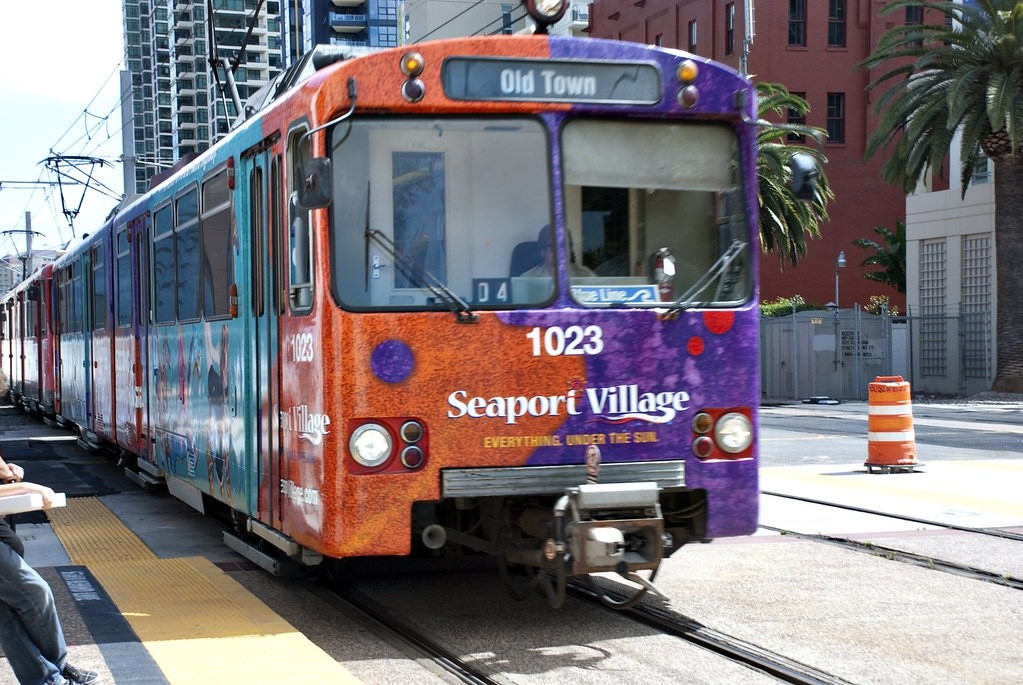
0 455 100 685
521 224 596 278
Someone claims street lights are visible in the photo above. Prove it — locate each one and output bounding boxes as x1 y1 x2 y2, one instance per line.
823 250 848 402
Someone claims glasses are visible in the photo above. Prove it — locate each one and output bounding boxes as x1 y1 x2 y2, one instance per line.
543 243 555 250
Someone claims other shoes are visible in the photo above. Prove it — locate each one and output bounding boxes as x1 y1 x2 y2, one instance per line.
64 663 100 685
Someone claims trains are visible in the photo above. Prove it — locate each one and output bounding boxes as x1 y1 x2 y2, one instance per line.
0 0 821 611
